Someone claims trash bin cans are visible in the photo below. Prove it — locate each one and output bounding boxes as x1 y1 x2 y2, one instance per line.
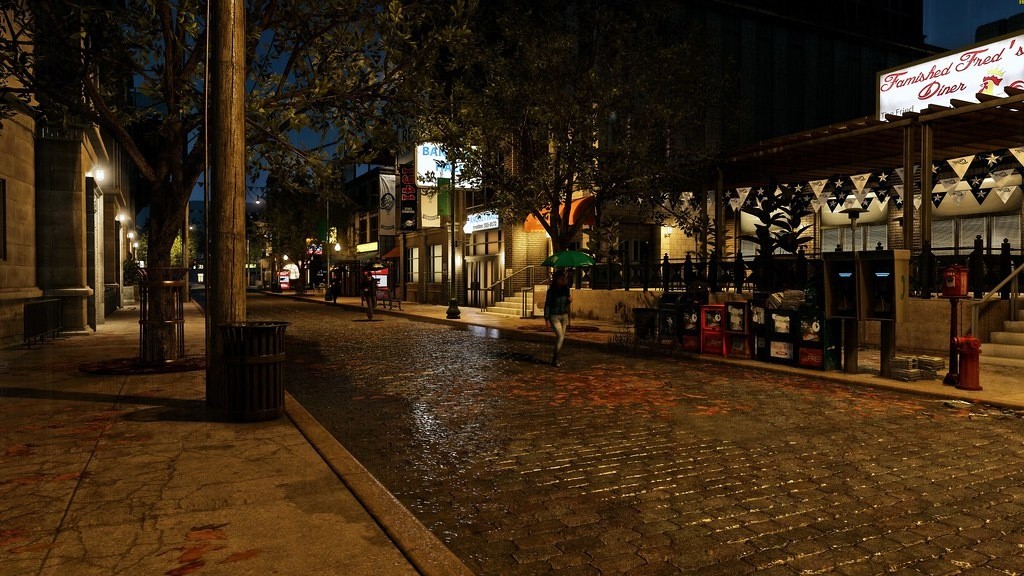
215 321 293 424
631 307 659 346
658 291 842 372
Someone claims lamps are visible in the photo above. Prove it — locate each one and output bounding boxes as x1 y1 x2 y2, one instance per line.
463 218 474 235
119 211 125 221
664 222 672 238
256 195 260 204
95 164 104 182
334 243 341 251
283 255 288 260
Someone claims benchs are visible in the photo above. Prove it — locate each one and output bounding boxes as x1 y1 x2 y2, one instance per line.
360 286 401 311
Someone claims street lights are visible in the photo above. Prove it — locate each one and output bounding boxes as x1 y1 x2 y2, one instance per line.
277 253 288 292
326 242 341 285
838 207 871 252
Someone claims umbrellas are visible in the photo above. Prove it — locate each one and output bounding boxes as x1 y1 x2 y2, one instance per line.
355 257 388 272
329 266 348 272
540 250 596 268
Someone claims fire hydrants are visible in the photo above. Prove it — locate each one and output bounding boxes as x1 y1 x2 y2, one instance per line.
952 334 984 390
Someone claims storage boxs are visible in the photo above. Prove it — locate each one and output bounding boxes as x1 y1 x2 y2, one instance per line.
632 291 838 371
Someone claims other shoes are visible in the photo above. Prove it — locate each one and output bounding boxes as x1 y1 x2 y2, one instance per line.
553 360 563 368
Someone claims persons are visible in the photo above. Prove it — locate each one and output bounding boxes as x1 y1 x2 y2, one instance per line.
361 271 377 320
330 273 340 303
545 268 572 366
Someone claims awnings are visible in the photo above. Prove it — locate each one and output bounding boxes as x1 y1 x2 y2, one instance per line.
381 246 400 258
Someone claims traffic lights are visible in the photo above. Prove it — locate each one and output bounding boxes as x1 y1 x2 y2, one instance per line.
193 261 197 270
